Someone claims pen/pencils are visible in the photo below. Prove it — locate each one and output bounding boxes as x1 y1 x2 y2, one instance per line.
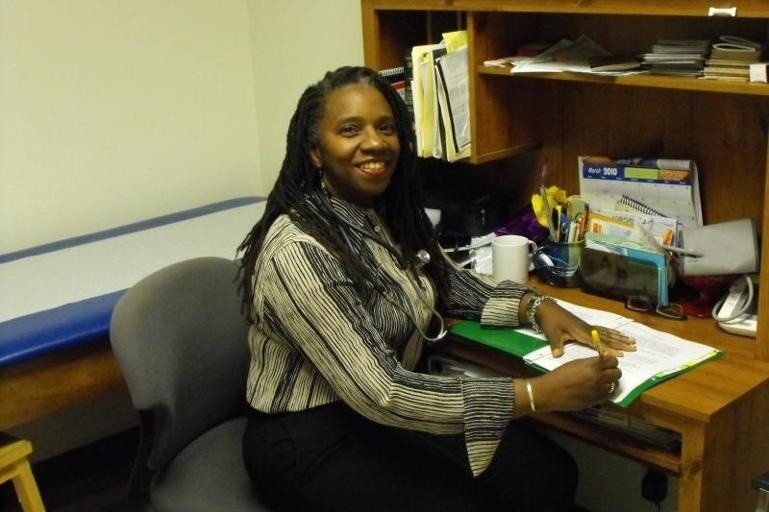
539 184 589 243
591 329 604 358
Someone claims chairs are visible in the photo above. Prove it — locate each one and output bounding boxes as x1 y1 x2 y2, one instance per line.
108 256 270 511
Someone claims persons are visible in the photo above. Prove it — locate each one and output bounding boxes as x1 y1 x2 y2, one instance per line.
232 67 637 512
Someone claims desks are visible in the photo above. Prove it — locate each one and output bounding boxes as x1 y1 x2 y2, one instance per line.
416 277 769 512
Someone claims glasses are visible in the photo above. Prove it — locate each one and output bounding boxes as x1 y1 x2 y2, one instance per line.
625 295 688 321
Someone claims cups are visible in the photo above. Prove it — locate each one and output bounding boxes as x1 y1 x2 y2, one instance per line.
491 235 538 288
536 240 591 287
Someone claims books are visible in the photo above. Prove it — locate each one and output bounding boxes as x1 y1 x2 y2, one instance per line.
641 35 763 81
614 194 684 238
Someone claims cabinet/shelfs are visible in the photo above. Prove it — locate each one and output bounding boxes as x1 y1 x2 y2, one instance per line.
359 0 768 364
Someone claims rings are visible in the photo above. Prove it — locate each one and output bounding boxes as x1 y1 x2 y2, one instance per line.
608 382 615 393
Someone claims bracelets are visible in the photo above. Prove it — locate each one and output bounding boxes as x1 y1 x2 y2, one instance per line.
526 379 536 413
527 294 558 333
